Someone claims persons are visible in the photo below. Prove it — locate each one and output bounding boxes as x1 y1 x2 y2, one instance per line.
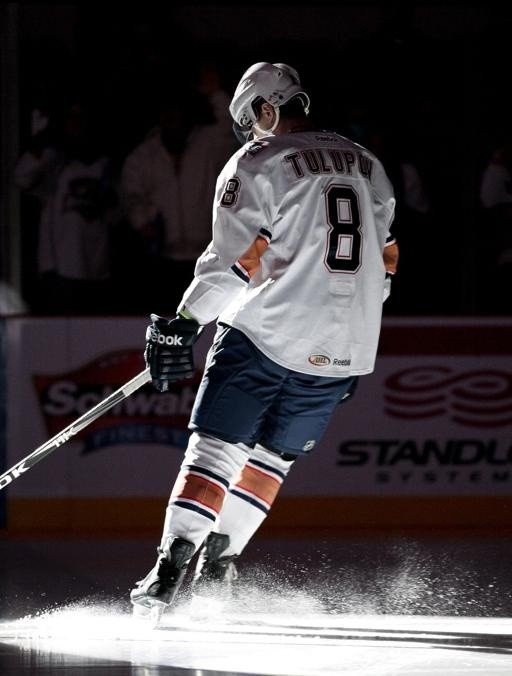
178 87 243 267
118 126 186 320
128 59 401 631
16 125 118 315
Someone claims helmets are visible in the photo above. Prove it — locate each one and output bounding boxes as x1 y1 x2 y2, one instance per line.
228 61 311 128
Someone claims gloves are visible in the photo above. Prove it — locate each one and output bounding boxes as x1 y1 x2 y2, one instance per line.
144 315 205 391
337 377 359 403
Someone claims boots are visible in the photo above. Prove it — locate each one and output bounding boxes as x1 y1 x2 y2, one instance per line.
193 533 239 595
129 547 192 606
